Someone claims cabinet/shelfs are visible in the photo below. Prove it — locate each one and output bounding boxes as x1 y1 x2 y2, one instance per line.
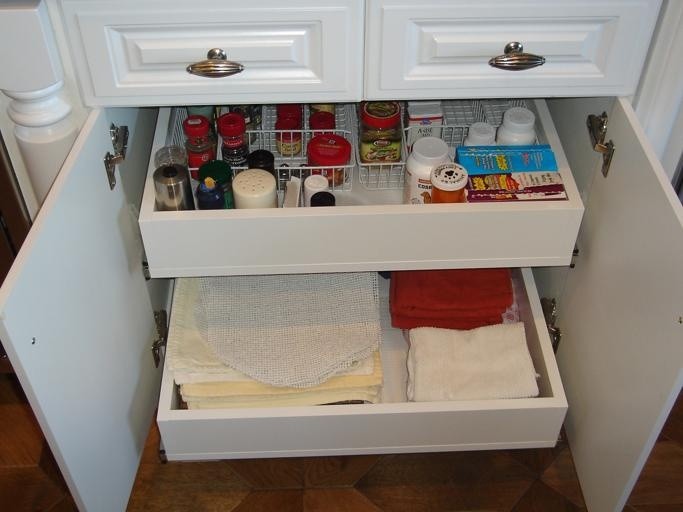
0 0 683 511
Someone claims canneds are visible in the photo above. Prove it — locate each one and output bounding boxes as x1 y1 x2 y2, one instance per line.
359 100 401 162
275 104 303 155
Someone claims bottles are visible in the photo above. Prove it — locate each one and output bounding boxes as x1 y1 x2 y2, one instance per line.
462 122 496 146
402 136 452 203
303 174 336 207
152 104 304 212
497 106 537 146
